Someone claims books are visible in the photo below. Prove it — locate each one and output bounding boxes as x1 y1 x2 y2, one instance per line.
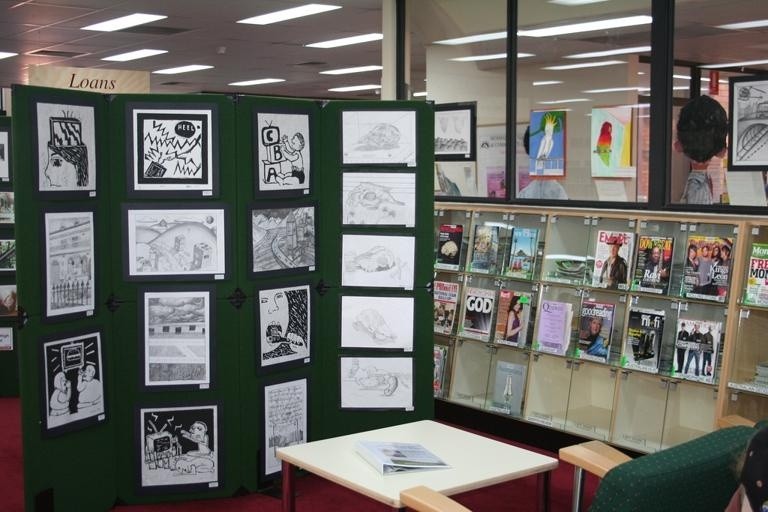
673 321 724 387
576 299 621 366
746 361 768 390
491 361 526 417
544 253 594 285
623 308 672 375
433 284 578 358
741 243 768 307
433 344 453 398
433 225 539 280
592 230 735 304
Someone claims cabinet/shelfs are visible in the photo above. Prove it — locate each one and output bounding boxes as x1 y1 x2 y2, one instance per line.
433 194 768 458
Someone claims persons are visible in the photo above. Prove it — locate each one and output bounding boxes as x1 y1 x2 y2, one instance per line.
50 373 71 418
45 141 89 188
77 365 102 409
259 291 308 360
517 125 569 200
179 421 210 455
674 94 727 206
276 133 305 185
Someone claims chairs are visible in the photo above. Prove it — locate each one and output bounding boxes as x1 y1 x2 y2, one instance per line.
720 414 768 512
398 426 754 512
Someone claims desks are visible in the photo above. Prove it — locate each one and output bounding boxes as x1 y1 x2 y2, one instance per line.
276 420 559 512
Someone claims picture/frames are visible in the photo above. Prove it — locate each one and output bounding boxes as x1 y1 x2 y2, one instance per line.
124 101 220 198
259 375 311 484
137 283 217 393
39 207 99 325
134 400 223 496
254 280 315 376
252 105 314 199
246 200 320 280
337 106 420 411
727 75 768 171
39 325 109 440
121 201 232 282
0 126 19 355
31 94 101 201
433 101 476 161
